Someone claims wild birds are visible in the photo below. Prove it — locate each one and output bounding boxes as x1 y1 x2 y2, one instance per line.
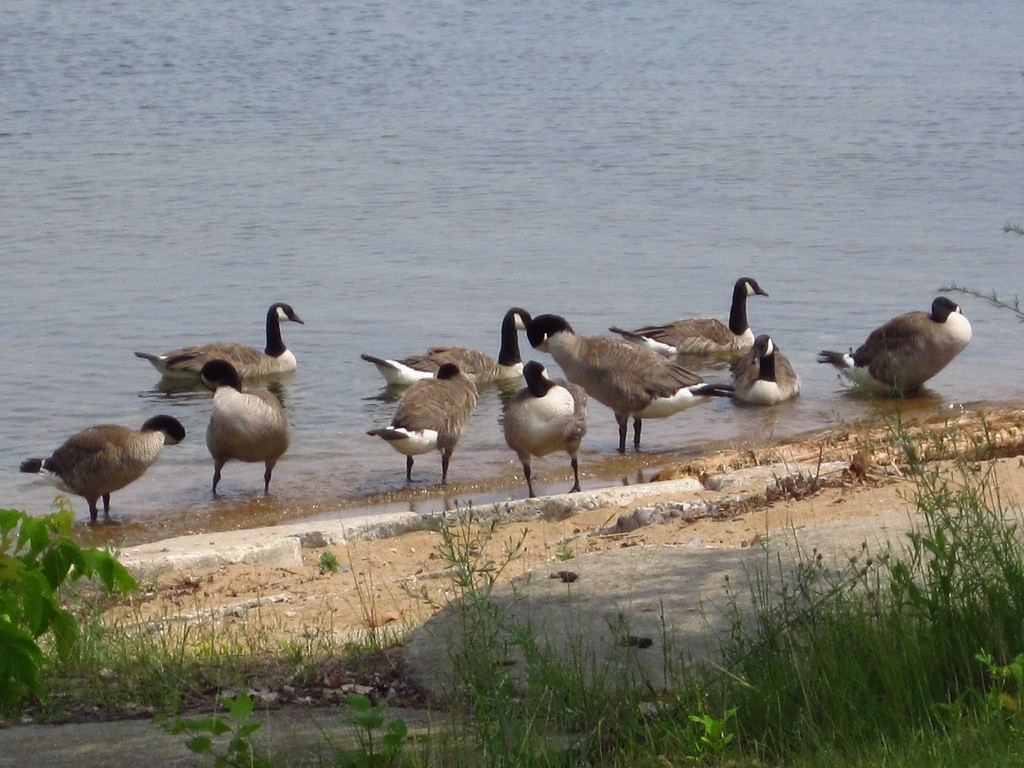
18 277 972 519
